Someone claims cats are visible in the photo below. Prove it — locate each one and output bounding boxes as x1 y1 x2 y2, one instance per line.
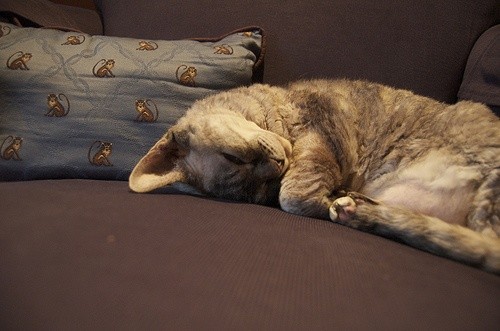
128 77 500 274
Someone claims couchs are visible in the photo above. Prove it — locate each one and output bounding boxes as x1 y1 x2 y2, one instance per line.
0 1 500 330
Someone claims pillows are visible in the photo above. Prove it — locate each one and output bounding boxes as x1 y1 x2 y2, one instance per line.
0 7 266 183
